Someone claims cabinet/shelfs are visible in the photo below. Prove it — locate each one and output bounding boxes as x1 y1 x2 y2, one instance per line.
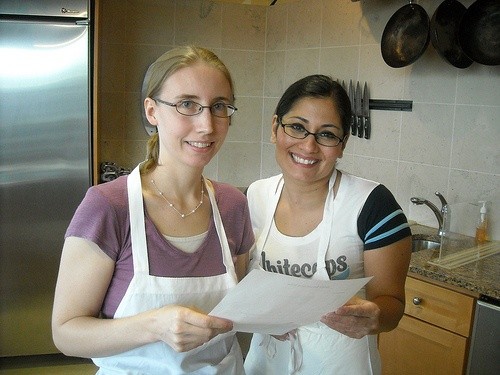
234 184 500 375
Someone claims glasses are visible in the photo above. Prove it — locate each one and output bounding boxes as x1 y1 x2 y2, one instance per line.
277 117 347 147
154 97 238 119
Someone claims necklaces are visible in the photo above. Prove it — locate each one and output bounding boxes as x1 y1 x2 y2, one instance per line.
147 174 204 217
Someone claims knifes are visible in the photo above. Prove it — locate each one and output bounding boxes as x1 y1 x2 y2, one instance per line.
356 81 363 138
364 81 371 139
336 78 357 136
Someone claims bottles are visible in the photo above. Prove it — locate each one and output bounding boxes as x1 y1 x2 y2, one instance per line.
475 200 488 240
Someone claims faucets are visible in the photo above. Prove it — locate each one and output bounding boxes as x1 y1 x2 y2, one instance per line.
410 190 449 237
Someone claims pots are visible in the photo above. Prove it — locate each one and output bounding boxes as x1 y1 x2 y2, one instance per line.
381 0 429 68
430 0 475 68
458 0 500 65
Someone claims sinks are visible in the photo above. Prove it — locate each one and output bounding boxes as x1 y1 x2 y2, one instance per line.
404 226 442 256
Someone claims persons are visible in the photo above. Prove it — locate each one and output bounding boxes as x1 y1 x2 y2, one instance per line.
243 75 411 375
52 45 256 375
